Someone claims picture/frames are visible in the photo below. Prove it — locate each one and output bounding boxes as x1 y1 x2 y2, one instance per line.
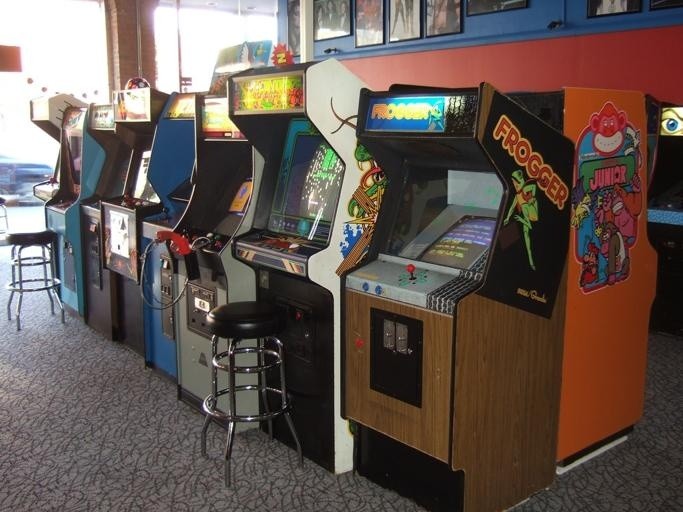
354 0 385 47
584 0 644 19
387 0 425 45
464 0 528 19
312 0 352 43
284 0 300 58
647 1 682 12
425 0 464 39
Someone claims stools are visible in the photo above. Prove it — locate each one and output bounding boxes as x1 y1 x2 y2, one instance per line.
3 228 69 330
195 297 310 488
0 197 11 233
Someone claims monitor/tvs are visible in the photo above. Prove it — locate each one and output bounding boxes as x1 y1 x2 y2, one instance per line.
66 130 254 236
282 131 345 223
416 215 497 269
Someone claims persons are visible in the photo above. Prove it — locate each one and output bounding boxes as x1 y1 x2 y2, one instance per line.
390 0 405 36
336 1 350 31
404 0 415 35
316 3 326 30
325 0 336 29
